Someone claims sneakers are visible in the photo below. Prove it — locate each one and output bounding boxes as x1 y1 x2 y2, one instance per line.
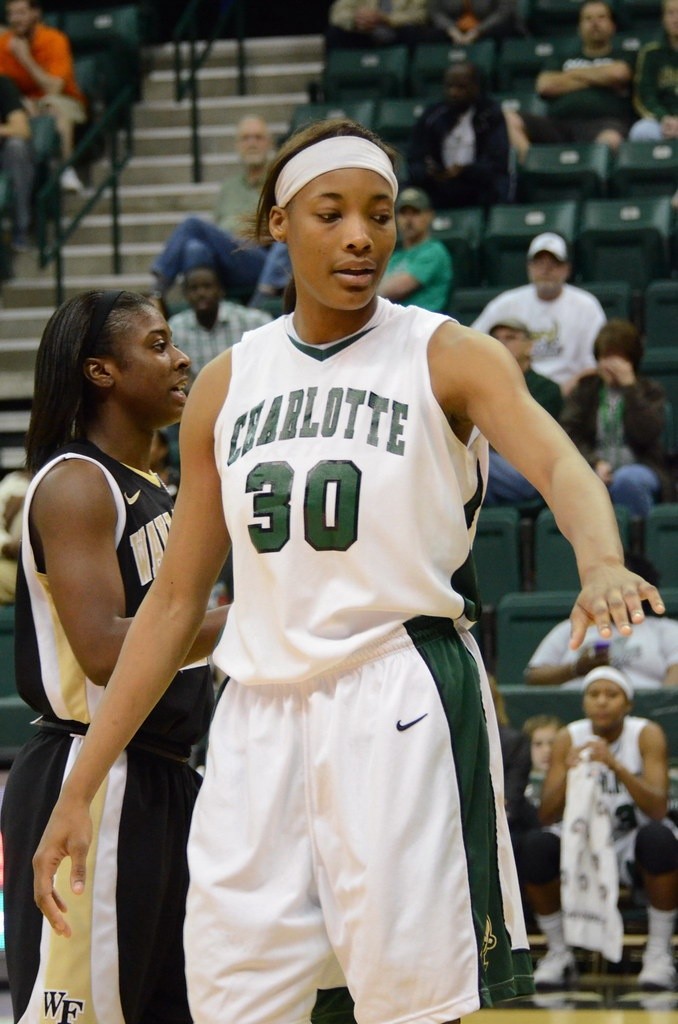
531 948 579 992
637 949 677 991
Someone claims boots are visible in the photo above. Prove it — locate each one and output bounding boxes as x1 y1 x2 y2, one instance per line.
629 519 659 588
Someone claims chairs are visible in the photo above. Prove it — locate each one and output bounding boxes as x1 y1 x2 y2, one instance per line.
27 8 142 144
287 0 677 1004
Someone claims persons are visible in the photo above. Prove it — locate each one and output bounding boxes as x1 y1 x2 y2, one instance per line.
31 118 666 1024
0 287 231 1024
0 0 678 991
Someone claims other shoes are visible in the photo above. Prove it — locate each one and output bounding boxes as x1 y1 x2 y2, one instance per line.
59 166 82 193
10 230 29 254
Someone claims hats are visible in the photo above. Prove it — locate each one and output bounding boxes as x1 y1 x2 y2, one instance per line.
526 231 568 264
395 186 431 211
